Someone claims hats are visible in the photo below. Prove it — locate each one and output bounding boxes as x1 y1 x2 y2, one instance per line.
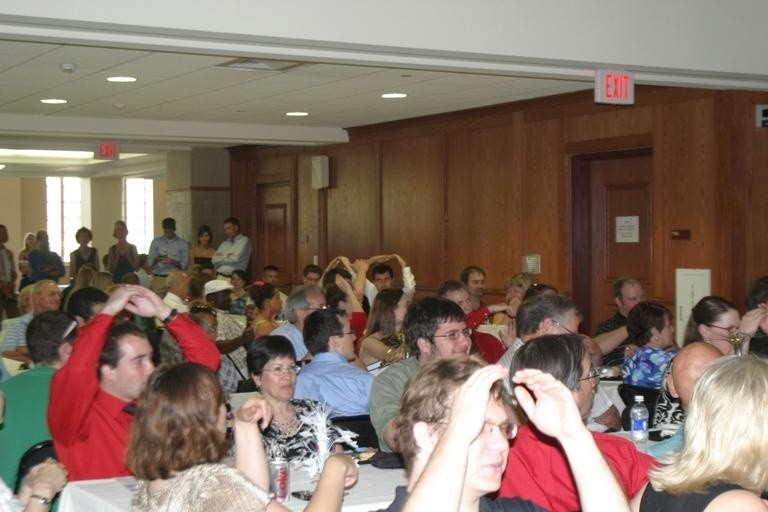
204 280 233 296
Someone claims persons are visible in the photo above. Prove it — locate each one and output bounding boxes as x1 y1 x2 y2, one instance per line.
0 215 767 511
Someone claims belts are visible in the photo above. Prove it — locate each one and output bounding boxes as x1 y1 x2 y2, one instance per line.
153 273 169 277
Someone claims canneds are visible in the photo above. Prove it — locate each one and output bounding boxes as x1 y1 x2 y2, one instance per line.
268 457 289 499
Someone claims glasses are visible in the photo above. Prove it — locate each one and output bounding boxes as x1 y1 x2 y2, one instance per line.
578 368 601 386
479 420 518 439
258 365 301 376
551 319 577 335
424 328 472 341
302 305 330 310
335 329 355 337
704 322 740 335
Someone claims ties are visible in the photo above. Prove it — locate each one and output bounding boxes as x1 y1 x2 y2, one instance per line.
124 406 136 415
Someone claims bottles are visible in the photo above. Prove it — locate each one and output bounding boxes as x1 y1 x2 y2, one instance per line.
630 396 649 452
